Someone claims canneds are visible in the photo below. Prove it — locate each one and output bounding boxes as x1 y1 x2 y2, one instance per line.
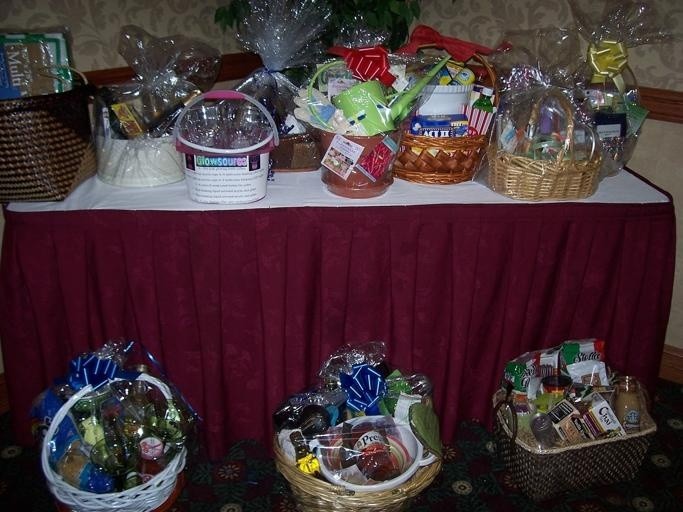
530 412 554 446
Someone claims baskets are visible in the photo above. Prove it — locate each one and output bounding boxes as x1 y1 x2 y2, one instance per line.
391 41 500 183
494 373 657 502
273 429 443 511
42 374 189 512
0 66 99 201
486 89 602 200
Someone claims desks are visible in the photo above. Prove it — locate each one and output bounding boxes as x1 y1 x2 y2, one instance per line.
2 75 676 460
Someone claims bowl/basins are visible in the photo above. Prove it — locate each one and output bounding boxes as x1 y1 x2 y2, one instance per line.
90 435 142 475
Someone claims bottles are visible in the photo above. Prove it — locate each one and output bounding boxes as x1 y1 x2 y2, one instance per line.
120 363 151 442
100 86 148 140
615 376 641 435
289 431 320 478
146 92 199 138
472 86 494 113
